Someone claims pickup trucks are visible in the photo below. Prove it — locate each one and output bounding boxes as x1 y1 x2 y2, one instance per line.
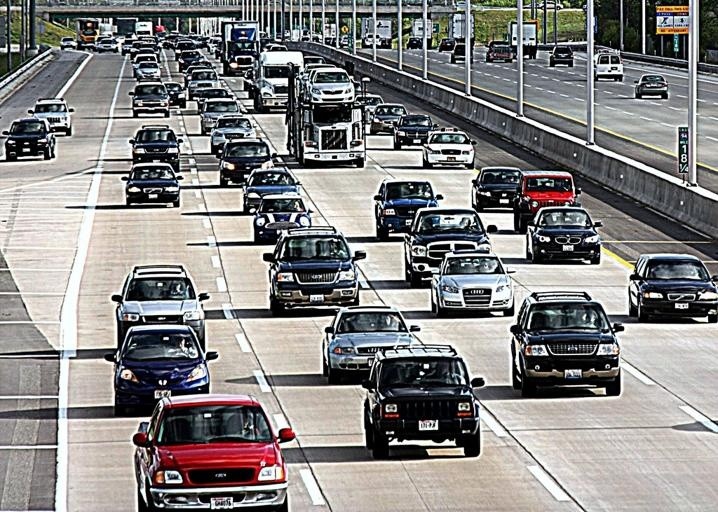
132 392 296 511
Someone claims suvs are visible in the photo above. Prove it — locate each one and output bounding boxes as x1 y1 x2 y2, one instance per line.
28 97 74 136
360 342 488 458
111 262 212 351
4 118 55 163
510 289 624 397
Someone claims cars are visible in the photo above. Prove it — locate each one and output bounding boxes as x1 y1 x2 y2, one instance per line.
626 251 718 324
103 324 219 418
320 303 420 384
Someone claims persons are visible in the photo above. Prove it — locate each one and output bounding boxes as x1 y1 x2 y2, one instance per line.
168 337 188 353
431 361 459 384
170 282 184 296
479 260 488 269
160 169 171 176
324 241 344 257
235 410 259 436
